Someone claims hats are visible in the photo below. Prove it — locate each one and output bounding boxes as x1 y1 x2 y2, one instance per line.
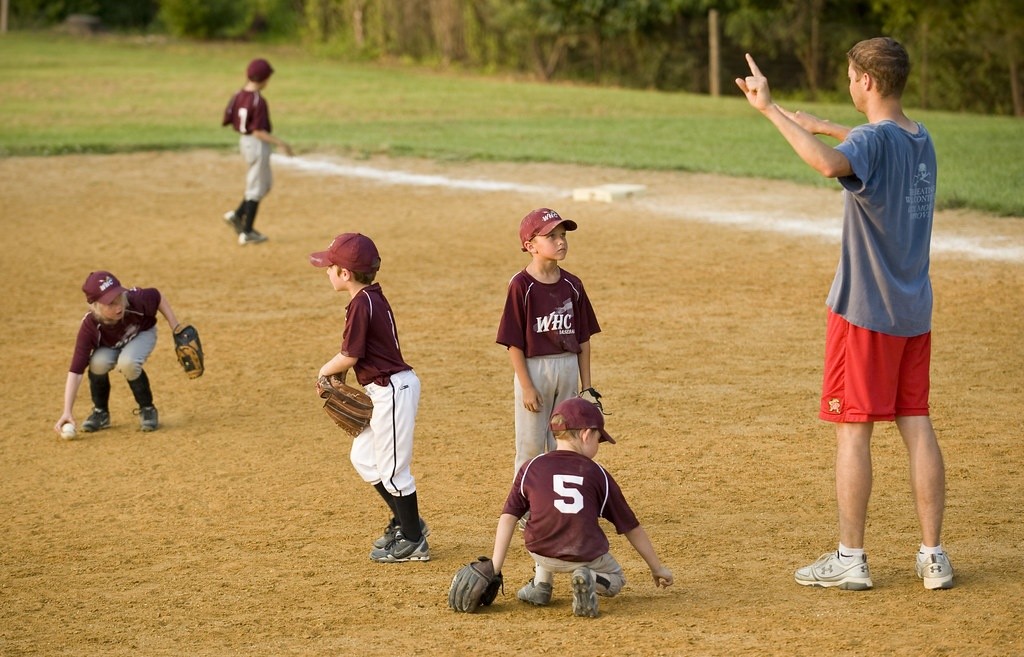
520 208 576 253
246 59 274 82
81 271 127 305
550 396 616 445
307 232 382 274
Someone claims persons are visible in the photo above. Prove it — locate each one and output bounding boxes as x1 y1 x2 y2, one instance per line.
736 36 959 591
221 59 294 246
309 233 431 562
446 398 674 617
54 271 203 431
495 208 600 529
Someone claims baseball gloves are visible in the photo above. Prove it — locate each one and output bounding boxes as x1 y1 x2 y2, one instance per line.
579 388 614 416
446 556 505 614
315 373 375 439
172 320 205 380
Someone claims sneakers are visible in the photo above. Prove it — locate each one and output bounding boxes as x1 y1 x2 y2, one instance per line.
795 550 874 590
375 518 428 548
133 405 157 432
370 535 429 562
570 568 598 617
916 552 953 590
515 577 552 605
81 406 111 432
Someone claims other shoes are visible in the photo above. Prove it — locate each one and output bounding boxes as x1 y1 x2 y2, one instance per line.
239 231 265 245
224 211 245 232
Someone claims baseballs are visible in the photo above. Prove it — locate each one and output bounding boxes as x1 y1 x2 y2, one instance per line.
60 422 77 440
